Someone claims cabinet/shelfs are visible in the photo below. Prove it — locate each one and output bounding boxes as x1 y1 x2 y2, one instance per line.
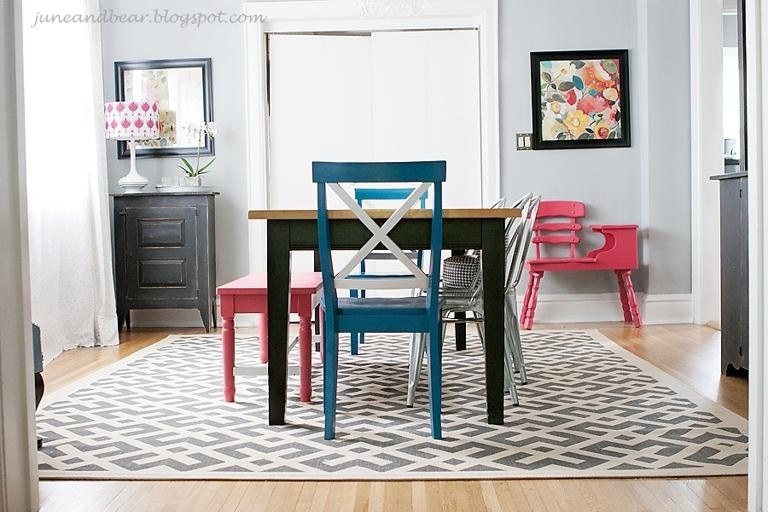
109 191 220 333
710 170 749 377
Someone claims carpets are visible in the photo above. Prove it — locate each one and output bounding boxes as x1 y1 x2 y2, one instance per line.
34 328 749 481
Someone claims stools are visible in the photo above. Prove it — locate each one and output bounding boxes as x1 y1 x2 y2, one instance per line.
216 272 324 403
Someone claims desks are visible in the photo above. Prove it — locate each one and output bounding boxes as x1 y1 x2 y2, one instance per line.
248 208 522 426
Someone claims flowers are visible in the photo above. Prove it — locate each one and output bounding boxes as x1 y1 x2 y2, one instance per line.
178 121 219 177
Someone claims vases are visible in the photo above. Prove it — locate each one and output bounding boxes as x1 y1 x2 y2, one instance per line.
187 176 201 187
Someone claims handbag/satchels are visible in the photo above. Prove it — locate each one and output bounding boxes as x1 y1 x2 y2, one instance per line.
443 255 480 289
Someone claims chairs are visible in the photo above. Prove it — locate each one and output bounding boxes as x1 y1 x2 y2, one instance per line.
520 200 641 331
311 160 542 439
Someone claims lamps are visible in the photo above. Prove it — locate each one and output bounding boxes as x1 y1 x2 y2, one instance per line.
104 100 160 194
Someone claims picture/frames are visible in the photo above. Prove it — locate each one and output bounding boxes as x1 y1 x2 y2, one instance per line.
114 58 215 159
530 49 631 150
